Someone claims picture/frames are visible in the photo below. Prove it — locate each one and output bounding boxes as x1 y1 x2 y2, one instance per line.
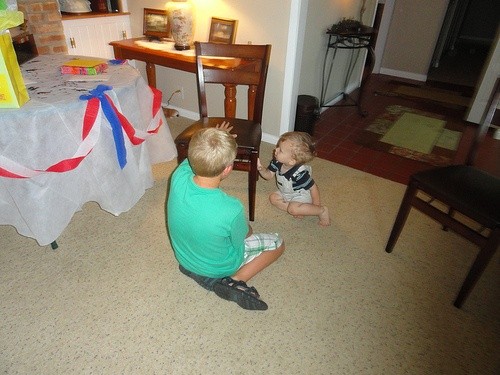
209 17 239 44
142 8 172 37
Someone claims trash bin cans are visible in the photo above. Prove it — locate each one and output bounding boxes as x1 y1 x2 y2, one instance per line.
293 94 316 135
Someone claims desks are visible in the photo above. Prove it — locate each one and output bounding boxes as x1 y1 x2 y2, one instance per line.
109 36 258 121
0 55 179 250
320 25 378 107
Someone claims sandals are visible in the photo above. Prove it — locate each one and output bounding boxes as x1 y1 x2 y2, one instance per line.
213 275 269 311
179 263 218 291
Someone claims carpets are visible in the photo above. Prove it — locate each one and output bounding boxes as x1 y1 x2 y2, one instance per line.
351 96 480 171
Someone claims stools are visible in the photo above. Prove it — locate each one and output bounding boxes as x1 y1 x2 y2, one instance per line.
385 164 500 309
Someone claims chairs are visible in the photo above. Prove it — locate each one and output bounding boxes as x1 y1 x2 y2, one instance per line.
175 42 272 221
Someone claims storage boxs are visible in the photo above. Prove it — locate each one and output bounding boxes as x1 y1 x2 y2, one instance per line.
0 32 31 108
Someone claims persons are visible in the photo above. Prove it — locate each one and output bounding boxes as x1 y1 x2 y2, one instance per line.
167 121 285 311
257 131 330 226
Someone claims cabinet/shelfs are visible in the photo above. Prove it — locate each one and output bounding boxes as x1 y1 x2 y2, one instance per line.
62 12 135 69
